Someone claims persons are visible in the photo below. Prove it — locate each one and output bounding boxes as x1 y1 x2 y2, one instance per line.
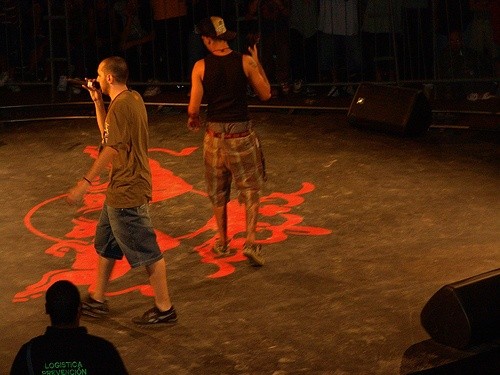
186 14 272 267
67 57 178 327
9 280 128 375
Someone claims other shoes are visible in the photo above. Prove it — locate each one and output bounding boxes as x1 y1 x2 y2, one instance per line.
249 91 257 98
304 97 320 104
143 79 162 95
466 92 479 101
56 74 68 91
214 238 231 255
0 72 8 86
291 78 304 93
241 240 265 267
72 77 82 93
271 88 279 99
478 91 496 100
279 79 290 92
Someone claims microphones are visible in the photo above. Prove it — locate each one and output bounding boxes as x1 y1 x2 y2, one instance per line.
63 78 100 89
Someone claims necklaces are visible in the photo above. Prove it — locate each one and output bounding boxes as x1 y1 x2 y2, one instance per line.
107 90 129 110
213 48 229 52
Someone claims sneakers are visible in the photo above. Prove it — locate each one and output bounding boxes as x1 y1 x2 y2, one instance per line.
80 292 112 317
132 305 177 326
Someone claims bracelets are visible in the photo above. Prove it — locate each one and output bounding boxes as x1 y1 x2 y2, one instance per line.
83 177 90 183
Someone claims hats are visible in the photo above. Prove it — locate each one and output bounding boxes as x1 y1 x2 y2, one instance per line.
198 16 237 42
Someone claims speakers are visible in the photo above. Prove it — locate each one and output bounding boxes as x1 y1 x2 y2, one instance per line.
418 268 500 351
347 83 434 140
